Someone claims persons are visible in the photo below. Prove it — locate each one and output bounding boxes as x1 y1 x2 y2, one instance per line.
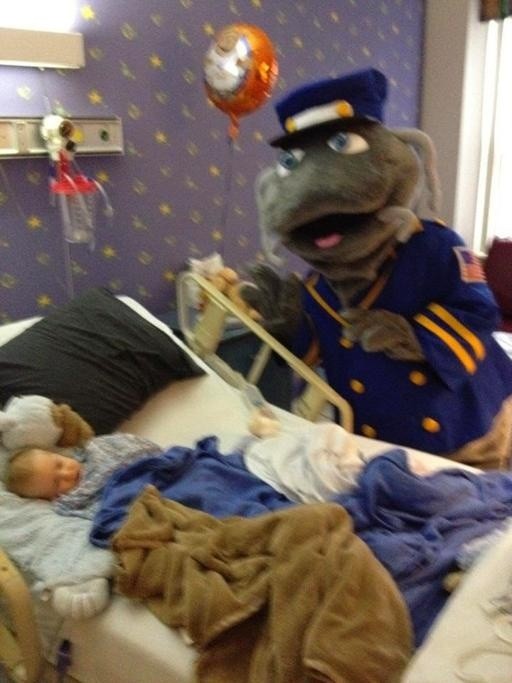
5 444 83 503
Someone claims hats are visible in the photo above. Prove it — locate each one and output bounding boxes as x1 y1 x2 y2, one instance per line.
268 68 389 147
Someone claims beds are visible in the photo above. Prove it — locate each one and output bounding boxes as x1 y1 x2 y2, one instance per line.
0 293 512 681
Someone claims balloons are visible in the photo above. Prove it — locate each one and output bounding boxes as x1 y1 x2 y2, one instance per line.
201 21 280 265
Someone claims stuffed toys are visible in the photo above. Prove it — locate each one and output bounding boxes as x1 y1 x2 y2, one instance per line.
237 67 511 472
181 253 255 318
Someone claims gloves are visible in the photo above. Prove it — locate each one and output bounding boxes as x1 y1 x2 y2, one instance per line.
341 308 422 363
240 264 310 344
